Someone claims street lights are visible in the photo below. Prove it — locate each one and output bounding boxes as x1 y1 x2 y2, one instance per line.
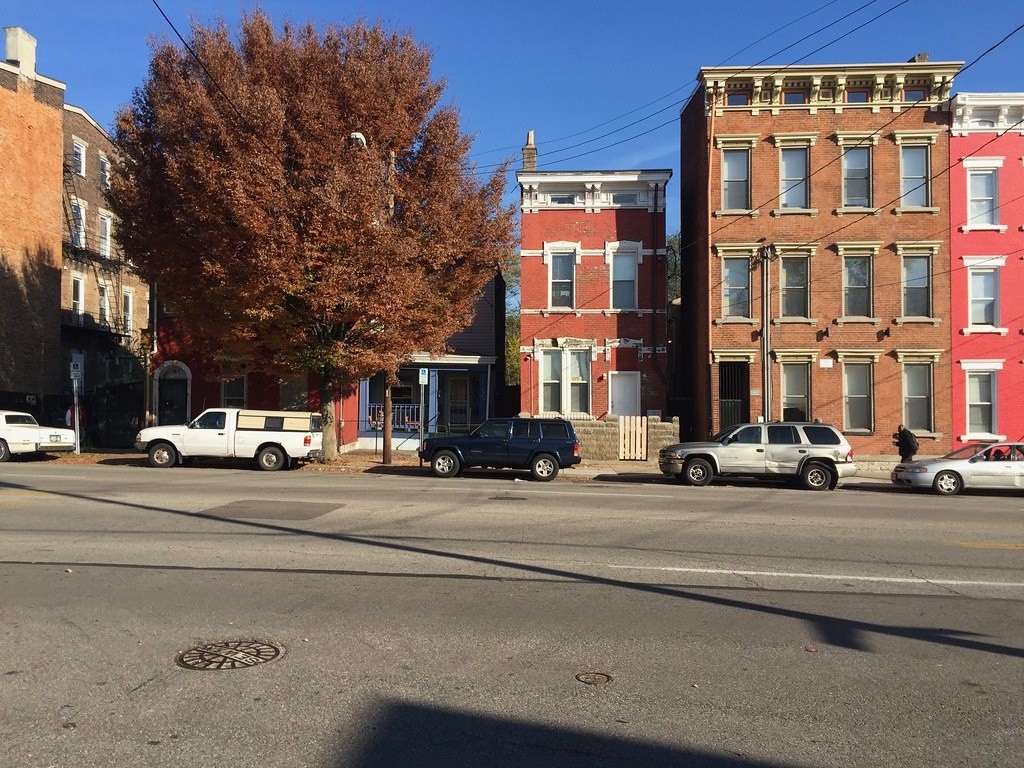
351 133 393 464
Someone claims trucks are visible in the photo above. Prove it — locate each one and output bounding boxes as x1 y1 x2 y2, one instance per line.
135 407 323 471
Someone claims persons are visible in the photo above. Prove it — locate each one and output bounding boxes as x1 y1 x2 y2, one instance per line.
992 449 1005 461
892 424 913 464
65 398 85 444
376 411 384 428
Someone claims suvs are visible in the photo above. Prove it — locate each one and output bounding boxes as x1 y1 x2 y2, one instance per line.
415 416 581 482
657 419 857 490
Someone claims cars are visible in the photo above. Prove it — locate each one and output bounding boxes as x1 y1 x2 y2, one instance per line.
890 440 1023 496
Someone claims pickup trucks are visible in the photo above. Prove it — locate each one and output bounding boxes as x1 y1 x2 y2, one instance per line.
0 409 77 463
